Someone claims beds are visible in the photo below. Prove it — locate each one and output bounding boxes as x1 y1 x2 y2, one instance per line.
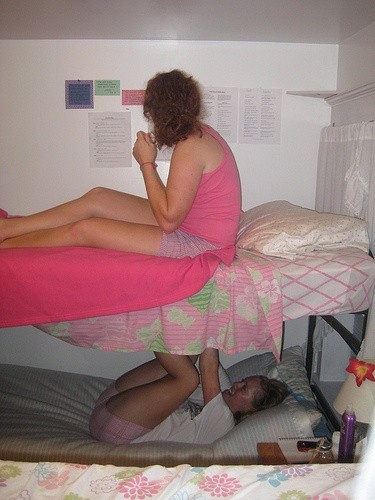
0 121 375 500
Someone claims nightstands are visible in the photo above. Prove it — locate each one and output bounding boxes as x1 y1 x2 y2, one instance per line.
255 434 356 466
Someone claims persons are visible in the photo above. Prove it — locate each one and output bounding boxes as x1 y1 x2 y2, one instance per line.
0 68 243 259
89 348 291 445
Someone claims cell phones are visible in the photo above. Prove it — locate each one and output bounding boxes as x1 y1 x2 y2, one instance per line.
297 440 330 452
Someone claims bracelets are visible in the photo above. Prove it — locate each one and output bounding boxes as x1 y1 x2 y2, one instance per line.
140 162 157 170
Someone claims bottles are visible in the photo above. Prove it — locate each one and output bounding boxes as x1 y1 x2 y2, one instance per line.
338 404 356 463
316 439 334 464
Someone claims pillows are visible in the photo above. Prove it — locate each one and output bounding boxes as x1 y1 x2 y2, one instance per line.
271 345 324 433
235 198 371 261
